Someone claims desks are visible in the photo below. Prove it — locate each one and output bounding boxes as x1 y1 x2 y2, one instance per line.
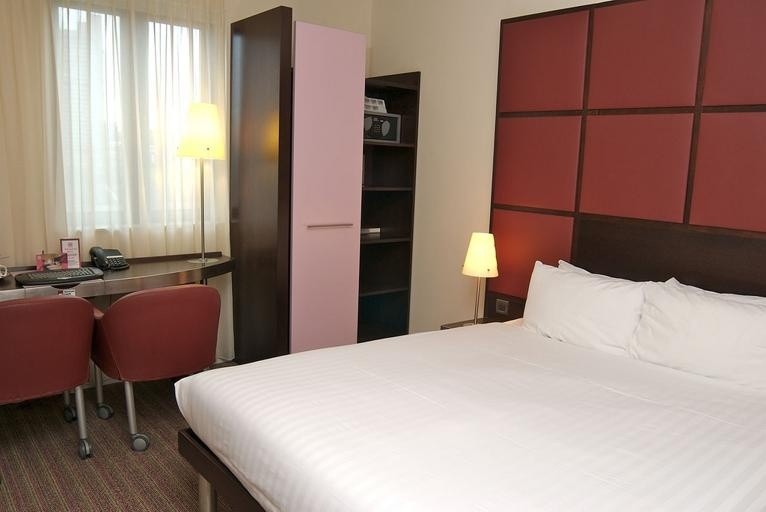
0 255 235 423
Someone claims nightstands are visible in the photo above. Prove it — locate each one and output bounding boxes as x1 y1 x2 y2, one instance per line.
441 318 505 331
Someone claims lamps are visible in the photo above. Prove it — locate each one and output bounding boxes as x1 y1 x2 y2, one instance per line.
463 232 499 325
179 103 226 262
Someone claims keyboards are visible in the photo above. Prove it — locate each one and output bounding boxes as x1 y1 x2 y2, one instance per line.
15 267 103 285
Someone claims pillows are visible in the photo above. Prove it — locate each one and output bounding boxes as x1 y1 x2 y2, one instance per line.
523 259 766 390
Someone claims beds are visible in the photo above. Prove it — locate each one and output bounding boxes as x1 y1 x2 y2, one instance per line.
174 214 765 511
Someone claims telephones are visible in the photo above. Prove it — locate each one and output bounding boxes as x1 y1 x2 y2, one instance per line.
89 247 130 271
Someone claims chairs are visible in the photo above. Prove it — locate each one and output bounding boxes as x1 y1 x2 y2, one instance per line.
1 297 95 458
91 284 221 450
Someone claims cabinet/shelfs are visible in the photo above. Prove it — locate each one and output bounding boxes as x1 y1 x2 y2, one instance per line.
357 72 422 343
